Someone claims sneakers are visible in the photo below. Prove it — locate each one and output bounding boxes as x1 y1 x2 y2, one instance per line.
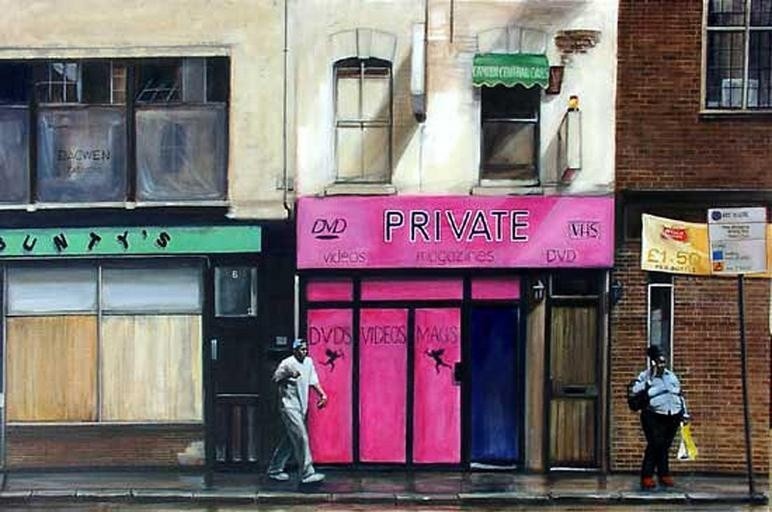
302 473 326 483
643 477 656 488
269 473 289 481
662 477 676 487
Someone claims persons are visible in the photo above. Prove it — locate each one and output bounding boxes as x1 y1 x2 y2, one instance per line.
265 338 328 484
632 344 691 487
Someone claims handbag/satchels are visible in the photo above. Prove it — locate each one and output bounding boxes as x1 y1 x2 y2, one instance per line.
627 379 650 411
677 420 699 462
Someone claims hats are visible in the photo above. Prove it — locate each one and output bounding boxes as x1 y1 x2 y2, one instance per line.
293 339 306 349
